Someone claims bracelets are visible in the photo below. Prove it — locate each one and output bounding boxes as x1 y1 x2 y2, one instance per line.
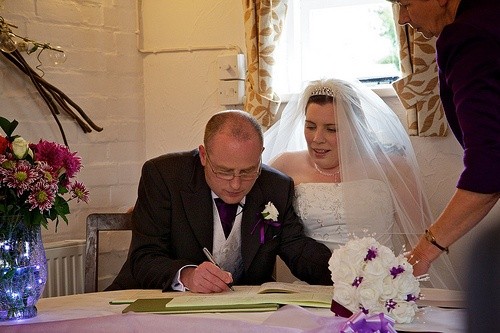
424 229 449 252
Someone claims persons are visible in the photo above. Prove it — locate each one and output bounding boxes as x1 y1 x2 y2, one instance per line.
108 110 332 294
127 77 461 290
389 0 500 277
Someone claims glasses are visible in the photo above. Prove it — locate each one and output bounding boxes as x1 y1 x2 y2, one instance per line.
204 150 263 181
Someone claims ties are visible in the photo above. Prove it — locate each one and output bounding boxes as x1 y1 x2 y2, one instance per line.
214 198 239 240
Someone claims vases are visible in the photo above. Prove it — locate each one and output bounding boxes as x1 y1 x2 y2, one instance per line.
0 224 47 320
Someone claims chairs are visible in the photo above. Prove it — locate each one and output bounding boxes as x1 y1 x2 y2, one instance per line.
85 210 133 294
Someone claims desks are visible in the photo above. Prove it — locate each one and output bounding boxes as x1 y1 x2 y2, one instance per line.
0 285 470 333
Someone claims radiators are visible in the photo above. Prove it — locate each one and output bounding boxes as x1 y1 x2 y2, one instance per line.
42 237 86 299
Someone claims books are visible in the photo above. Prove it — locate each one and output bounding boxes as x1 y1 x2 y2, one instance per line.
121 294 278 315
190 282 319 295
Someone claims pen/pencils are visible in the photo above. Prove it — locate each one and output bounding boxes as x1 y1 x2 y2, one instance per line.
203 247 236 291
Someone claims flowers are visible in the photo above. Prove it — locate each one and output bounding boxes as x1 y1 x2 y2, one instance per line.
0 116 92 281
328 230 432 324
250 202 281 235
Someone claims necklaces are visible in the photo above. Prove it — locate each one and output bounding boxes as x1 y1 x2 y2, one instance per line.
315 164 340 176
